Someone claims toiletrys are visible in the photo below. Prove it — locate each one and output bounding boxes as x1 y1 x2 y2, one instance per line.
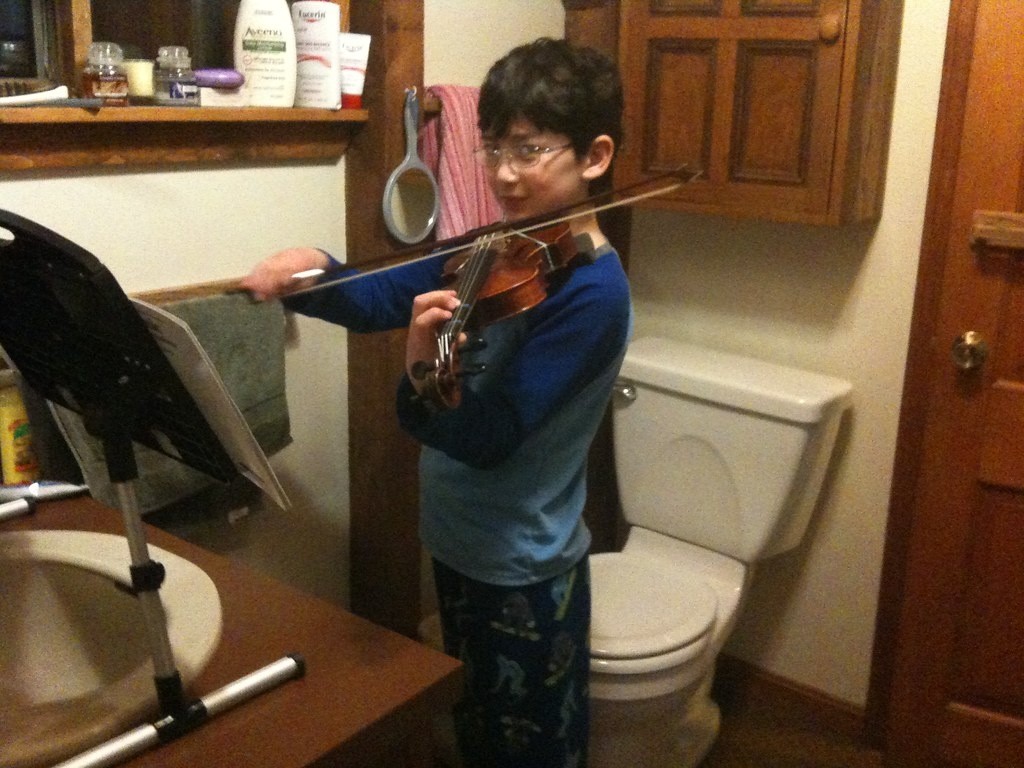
2 388 41 483
124 58 154 106
342 32 372 108
80 42 130 107
151 46 202 107
233 0 297 108
291 0 341 109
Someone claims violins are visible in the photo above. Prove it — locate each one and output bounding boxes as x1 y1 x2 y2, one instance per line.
411 215 579 411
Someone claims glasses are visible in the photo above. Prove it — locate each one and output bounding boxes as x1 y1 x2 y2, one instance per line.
472 141 577 170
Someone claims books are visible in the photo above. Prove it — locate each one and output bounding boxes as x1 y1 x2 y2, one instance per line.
0 298 292 510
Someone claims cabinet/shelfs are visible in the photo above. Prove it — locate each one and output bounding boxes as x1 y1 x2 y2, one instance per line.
611 0 905 227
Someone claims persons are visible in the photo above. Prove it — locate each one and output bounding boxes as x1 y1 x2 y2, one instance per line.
235 38 630 768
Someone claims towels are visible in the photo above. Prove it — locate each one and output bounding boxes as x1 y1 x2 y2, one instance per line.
423 82 505 244
50 287 293 518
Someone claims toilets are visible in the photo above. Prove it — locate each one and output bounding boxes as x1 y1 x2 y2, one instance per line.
578 333 855 761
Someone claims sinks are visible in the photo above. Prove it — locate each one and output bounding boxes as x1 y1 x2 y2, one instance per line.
2 531 224 763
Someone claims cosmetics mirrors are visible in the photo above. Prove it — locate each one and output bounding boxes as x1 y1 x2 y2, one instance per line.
383 93 440 244
